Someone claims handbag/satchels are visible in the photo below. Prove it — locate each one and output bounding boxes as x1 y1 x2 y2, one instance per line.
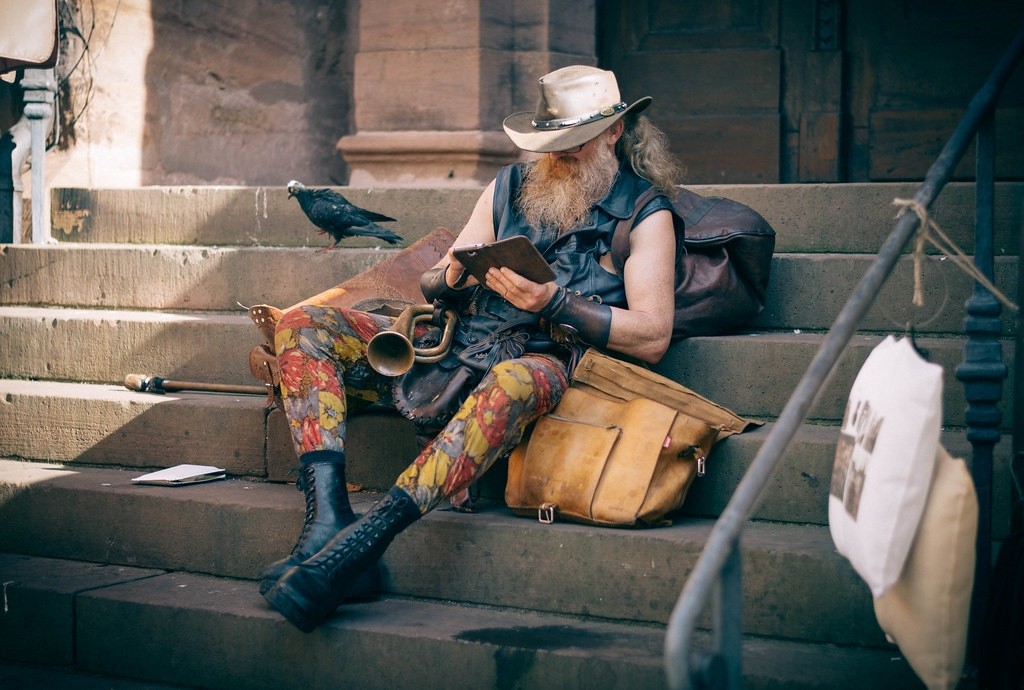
504 347 765 529
249 226 458 416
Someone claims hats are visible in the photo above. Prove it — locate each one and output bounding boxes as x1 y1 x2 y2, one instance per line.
503 64 653 153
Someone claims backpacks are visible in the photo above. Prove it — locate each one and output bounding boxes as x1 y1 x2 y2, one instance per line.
610 185 777 346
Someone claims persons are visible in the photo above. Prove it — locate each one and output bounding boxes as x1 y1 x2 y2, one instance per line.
258 64 686 634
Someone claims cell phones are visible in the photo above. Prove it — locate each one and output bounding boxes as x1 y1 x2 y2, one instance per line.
452 236 558 289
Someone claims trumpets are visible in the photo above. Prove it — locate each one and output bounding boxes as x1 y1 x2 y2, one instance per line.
368 299 460 377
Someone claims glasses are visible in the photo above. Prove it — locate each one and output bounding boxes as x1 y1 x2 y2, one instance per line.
560 143 586 153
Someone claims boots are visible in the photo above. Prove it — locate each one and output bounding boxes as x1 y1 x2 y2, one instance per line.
259 460 413 633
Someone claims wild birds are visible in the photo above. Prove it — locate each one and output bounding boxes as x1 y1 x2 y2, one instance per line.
288 180 404 255
